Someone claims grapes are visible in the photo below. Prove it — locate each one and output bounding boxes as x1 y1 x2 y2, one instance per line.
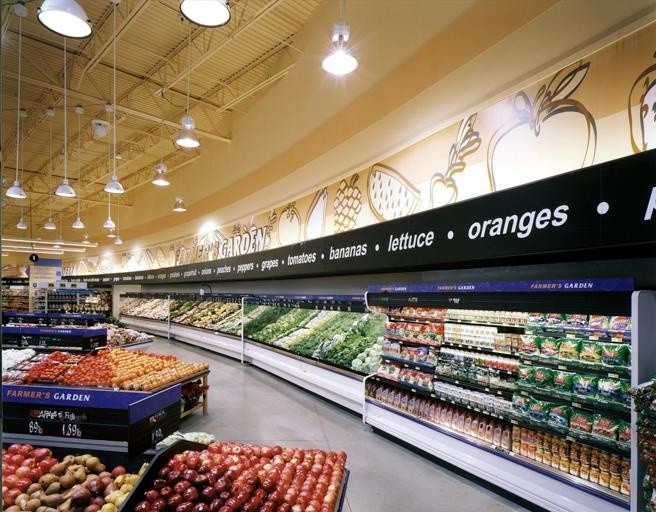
333 173 362 233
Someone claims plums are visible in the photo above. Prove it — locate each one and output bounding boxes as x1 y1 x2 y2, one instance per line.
133 440 347 512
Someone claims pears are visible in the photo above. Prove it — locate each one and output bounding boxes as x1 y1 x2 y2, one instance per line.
4 454 149 512
304 188 328 239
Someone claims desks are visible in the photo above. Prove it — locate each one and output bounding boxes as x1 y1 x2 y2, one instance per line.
1 320 350 511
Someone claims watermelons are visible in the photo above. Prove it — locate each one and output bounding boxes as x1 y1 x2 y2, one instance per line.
367 163 421 221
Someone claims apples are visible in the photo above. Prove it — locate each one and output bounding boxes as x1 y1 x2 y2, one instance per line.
2 443 58 509
487 60 597 193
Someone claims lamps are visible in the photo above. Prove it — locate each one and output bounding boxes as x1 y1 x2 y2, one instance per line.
72 105 86 230
176 17 201 149
4 16 27 200
104 102 115 229
180 0 231 28
15 106 28 230
173 164 186 213
323 1 357 75
150 86 171 188
113 151 123 246
43 106 57 230
37 1 93 40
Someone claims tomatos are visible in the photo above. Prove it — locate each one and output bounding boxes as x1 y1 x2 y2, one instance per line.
61 349 116 386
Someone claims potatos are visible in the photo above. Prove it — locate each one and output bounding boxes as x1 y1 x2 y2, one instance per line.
110 348 209 390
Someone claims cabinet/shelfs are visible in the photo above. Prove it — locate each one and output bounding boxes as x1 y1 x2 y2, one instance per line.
2 278 113 320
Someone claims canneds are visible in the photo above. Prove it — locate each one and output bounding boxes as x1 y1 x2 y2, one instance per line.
511 425 630 496
